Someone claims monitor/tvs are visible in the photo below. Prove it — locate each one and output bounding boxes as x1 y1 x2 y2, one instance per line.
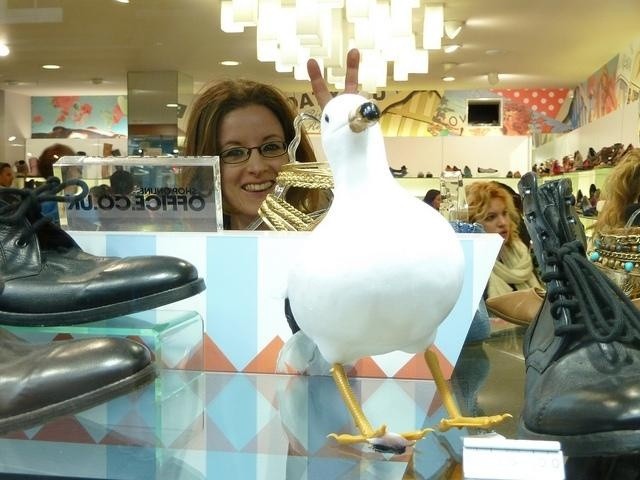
466 98 504 129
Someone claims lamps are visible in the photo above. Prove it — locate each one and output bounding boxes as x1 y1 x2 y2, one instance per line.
442 19 464 40
219 0 444 96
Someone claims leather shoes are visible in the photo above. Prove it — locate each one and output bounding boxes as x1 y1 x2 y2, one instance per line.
389 164 522 178
0 177 208 327
575 184 601 217
532 143 633 177
1 325 160 436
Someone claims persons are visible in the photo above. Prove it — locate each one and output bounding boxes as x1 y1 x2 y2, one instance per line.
181 48 359 231
463 182 548 318
0 144 133 196
423 189 441 212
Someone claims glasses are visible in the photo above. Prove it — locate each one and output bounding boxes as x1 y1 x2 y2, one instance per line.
219 139 289 164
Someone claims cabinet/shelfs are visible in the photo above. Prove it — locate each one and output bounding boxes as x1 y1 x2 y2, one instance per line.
394 165 619 253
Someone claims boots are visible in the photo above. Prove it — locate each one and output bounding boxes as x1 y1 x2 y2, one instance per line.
517 172 640 457
